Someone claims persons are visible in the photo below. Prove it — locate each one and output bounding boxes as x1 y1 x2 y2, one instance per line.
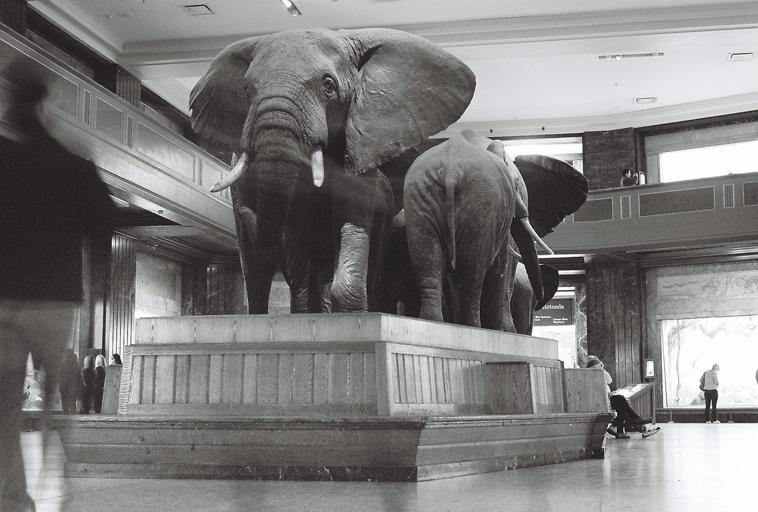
586 354 661 439
59 348 121 413
699 364 720 424
0 56 116 512
620 168 638 187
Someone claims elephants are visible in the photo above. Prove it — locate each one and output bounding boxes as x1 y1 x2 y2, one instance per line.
509 251 561 335
189 26 476 314
387 135 589 328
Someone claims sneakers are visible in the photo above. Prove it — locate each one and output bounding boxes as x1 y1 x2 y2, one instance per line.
607 427 616 436
713 420 721 424
641 426 661 440
616 433 630 442
706 420 711 424
